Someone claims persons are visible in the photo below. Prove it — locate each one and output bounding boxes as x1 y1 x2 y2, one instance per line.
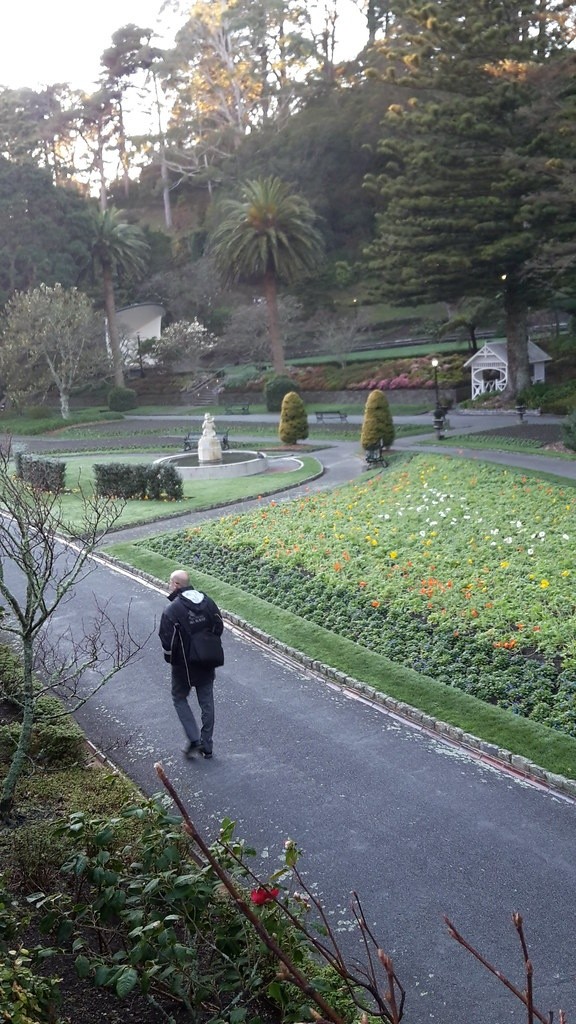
202 412 216 437
158 569 224 759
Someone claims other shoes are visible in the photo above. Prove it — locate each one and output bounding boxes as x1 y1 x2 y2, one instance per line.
200 746 213 759
181 741 202 755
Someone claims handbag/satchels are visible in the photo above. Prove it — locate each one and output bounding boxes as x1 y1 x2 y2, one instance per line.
183 629 224 668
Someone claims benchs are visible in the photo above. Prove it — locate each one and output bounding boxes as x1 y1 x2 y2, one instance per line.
183 428 230 452
224 402 250 415
314 411 348 425
